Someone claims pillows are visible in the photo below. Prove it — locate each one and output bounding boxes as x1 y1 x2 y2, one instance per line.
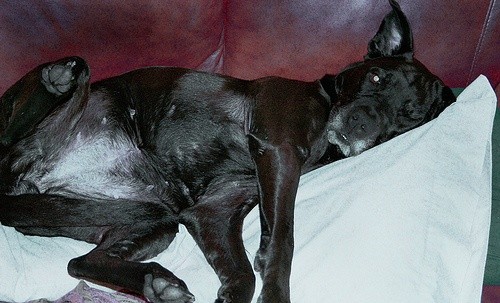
1 75 497 303
446 85 500 288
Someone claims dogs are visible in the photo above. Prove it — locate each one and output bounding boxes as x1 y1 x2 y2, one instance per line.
0 1 457 303
